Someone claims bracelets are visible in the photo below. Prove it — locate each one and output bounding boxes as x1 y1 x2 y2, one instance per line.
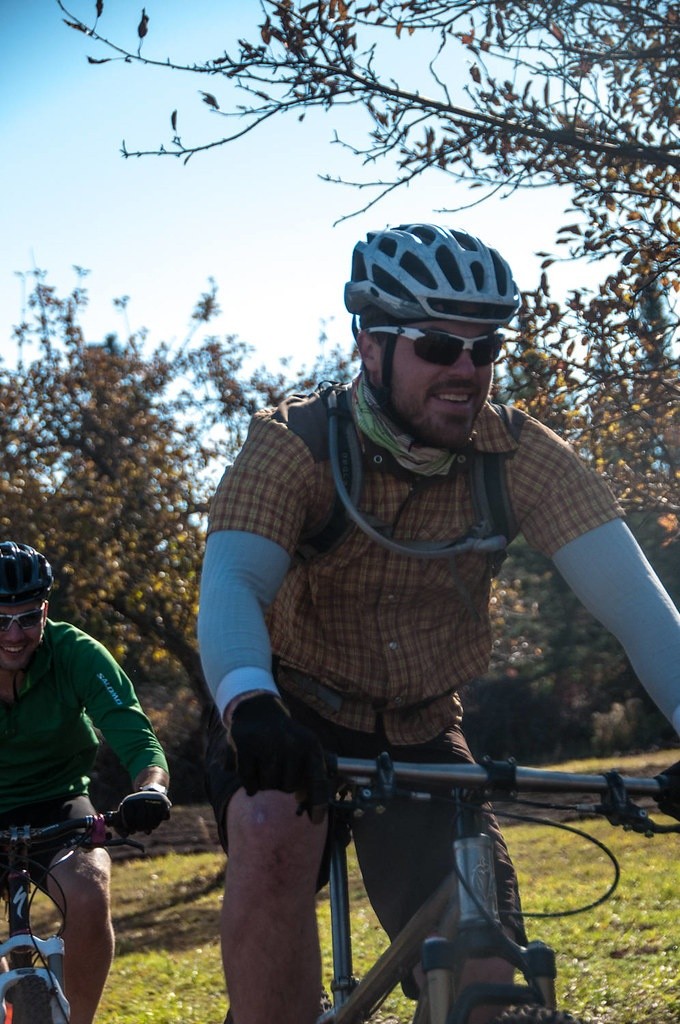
140 784 166 794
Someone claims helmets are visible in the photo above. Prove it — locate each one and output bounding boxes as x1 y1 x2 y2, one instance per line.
343 222 522 327
0 541 53 606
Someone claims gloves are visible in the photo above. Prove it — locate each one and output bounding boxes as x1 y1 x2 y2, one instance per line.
230 694 307 797
113 788 170 840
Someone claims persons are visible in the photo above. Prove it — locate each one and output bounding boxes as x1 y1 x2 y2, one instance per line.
194 222 680 1024
0 540 170 1024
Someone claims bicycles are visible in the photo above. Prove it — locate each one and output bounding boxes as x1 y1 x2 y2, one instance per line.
1 800 175 1024
224 750 675 1024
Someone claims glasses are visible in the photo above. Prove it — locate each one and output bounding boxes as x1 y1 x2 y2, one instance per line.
0 608 45 631
359 324 505 369
